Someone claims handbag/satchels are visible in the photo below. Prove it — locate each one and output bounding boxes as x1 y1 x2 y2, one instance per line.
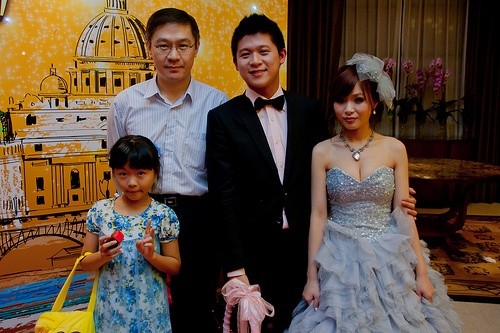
34 251 99 333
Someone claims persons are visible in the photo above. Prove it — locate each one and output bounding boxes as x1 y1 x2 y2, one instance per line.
285 52 462 333
81 134 180 333
204 13 417 333
107 6 229 333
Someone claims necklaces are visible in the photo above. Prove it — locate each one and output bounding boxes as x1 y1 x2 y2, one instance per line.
340 130 375 161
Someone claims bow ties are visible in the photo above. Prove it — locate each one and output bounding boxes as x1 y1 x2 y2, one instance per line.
254 95 285 110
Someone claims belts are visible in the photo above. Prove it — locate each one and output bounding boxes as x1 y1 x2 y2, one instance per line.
161 194 199 207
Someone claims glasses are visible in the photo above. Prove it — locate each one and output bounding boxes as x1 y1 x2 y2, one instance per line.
152 43 196 54
